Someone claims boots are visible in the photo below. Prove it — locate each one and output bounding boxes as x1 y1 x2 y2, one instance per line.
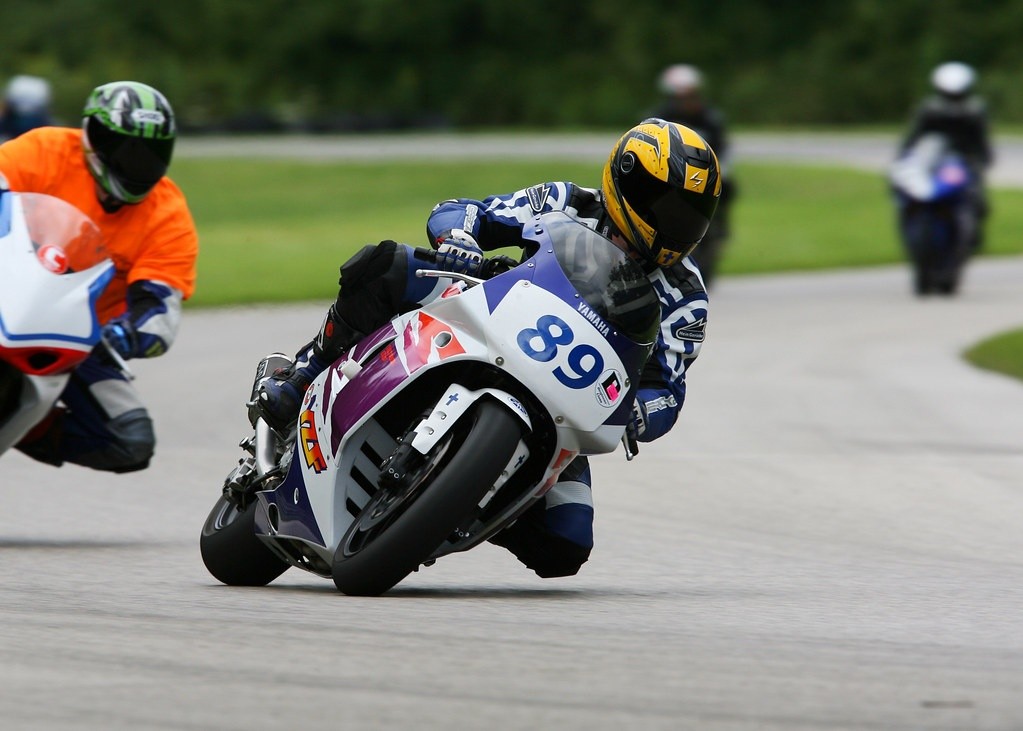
258 300 365 432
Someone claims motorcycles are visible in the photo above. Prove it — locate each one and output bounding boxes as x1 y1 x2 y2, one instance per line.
198 209 663 598
883 131 980 294
0 190 130 459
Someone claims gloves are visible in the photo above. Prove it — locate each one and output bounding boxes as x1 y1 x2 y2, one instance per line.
435 229 484 273
625 394 649 440
103 317 139 360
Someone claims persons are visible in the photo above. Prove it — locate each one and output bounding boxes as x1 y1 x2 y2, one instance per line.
266 116 724 580
0 71 200 475
654 65 738 248
894 48 995 233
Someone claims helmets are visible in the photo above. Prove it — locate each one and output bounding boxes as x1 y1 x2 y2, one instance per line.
657 63 707 98
601 117 722 270
81 80 177 204
6 74 51 117
930 61 979 99
